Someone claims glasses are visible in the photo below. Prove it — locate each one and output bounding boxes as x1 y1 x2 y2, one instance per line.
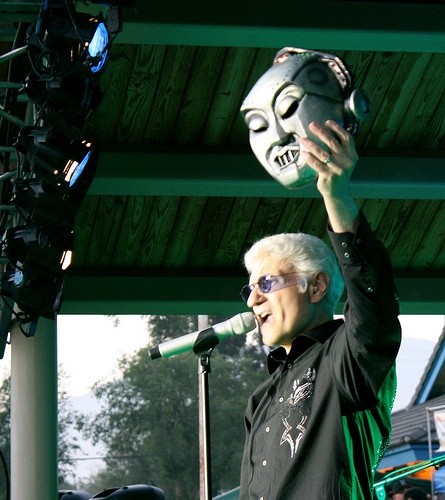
239 271 309 303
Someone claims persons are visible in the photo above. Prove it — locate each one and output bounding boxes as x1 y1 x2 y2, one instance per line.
389 487 428 500
238 119 402 500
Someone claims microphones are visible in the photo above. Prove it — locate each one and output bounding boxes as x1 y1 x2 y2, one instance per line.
149 312 256 359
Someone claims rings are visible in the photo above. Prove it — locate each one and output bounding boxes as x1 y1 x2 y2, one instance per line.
324 154 334 164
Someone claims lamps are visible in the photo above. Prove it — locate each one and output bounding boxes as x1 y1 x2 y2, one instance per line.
0 0 108 338
